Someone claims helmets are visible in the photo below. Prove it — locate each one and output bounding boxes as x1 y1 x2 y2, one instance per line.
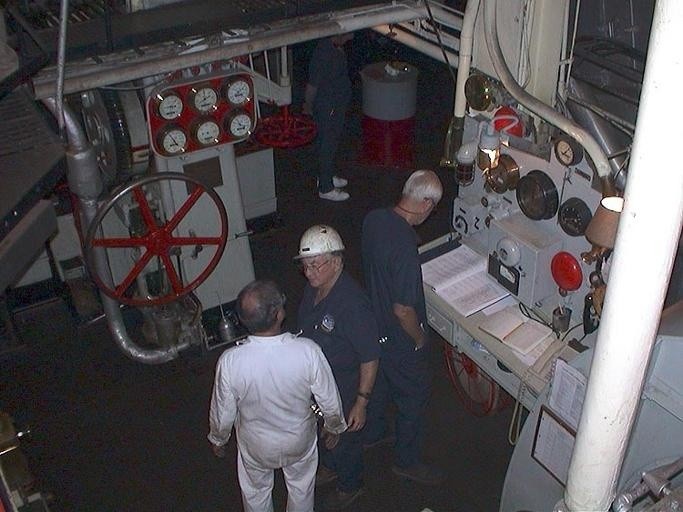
291 222 346 259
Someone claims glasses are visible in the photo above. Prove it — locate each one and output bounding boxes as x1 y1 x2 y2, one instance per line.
295 256 337 274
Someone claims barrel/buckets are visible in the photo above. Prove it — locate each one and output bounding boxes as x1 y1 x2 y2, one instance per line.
360 61 418 122
361 114 417 170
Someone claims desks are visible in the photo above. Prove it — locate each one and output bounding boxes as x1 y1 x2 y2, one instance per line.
418 231 581 412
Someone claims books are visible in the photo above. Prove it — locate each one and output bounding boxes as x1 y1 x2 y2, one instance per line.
478 305 554 355
420 244 511 317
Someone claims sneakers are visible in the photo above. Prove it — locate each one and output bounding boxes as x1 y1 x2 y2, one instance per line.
318 186 351 202
390 460 450 487
322 484 368 511
316 175 349 187
316 463 340 488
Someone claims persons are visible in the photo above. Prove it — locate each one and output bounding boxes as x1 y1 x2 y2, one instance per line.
292 225 384 511
359 168 450 486
299 31 356 202
206 279 350 512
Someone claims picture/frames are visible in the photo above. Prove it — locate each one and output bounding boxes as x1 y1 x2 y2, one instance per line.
530 404 577 489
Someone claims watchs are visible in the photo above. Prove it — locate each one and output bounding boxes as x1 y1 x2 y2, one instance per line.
356 392 372 401
